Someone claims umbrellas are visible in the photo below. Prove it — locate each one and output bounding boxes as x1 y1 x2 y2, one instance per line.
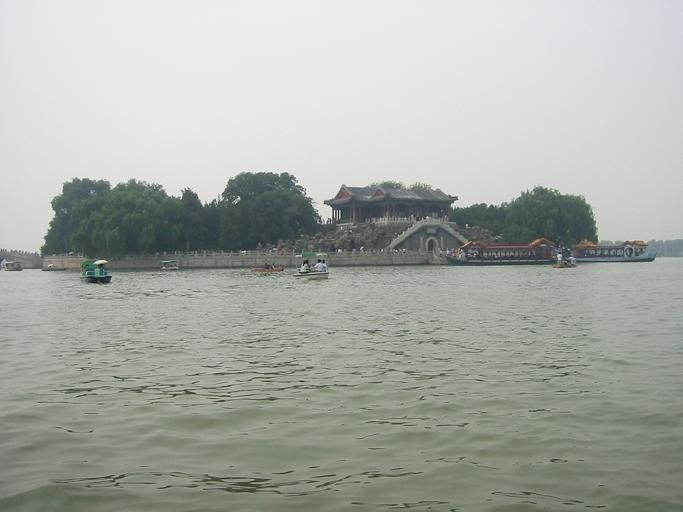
92 258 109 265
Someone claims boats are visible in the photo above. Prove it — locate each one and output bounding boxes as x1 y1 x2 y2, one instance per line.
293 252 329 279
252 268 284 272
445 238 557 264
552 262 577 269
161 260 179 270
5 261 24 271
79 260 112 283
42 269 66 271
571 239 659 262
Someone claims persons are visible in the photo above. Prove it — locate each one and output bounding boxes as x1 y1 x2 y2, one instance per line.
564 255 575 265
556 251 562 264
337 246 342 257
437 245 477 261
582 251 623 256
97 265 105 275
358 245 408 255
299 260 309 274
313 259 321 271
317 259 327 272
551 253 557 264
160 262 176 269
